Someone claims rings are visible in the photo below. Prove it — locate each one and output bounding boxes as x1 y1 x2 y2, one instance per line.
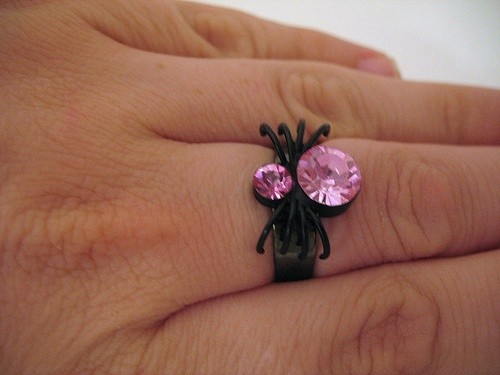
257 118 362 285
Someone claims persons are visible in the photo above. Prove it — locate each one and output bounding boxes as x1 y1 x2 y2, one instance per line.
1 0 500 375
